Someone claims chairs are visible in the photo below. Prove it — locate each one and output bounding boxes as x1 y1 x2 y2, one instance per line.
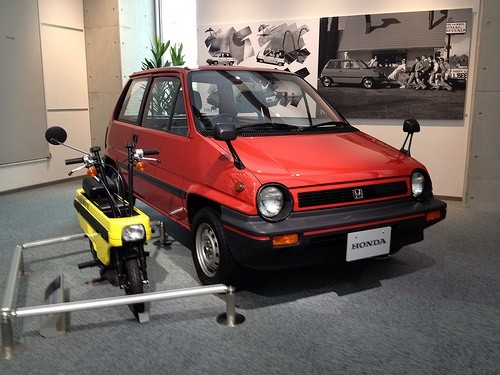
158 92 206 133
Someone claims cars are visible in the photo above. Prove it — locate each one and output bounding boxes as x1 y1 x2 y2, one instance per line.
103 67 447 286
255 49 287 67
319 59 386 89
206 53 235 66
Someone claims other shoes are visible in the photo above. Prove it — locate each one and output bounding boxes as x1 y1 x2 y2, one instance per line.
400 85 405 88
385 86 390 88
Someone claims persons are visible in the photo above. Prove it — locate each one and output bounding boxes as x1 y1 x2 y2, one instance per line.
367 55 382 67
406 55 453 91
387 57 407 89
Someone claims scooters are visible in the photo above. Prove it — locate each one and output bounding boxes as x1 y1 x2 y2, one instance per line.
46 126 162 313
